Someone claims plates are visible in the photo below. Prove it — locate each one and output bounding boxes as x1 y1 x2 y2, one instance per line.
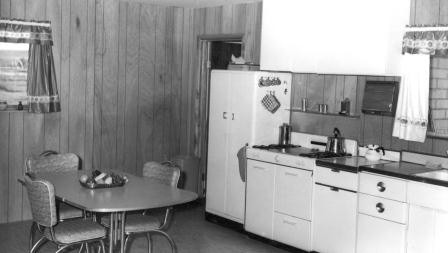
80 176 125 189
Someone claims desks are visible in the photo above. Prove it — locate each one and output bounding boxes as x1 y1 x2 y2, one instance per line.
33 168 198 252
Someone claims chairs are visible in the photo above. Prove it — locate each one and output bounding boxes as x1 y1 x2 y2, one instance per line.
24 172 107 253
117 162 181 252
30 151 93 252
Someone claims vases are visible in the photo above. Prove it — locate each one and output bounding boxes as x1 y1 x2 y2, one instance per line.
1 101 8 111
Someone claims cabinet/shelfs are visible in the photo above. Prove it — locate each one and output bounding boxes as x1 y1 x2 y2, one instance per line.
359 167 407 252
243 145 315 252
314 160 358 252
286 108 359 122
409 176 448 252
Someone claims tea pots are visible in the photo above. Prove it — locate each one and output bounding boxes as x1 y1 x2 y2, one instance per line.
365 143 385 161
310 128 347 156
278 122 293 146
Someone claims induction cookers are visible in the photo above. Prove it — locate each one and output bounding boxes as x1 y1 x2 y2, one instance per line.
248 143 353 162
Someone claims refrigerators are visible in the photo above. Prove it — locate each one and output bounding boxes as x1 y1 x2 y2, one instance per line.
204 68 293 232
